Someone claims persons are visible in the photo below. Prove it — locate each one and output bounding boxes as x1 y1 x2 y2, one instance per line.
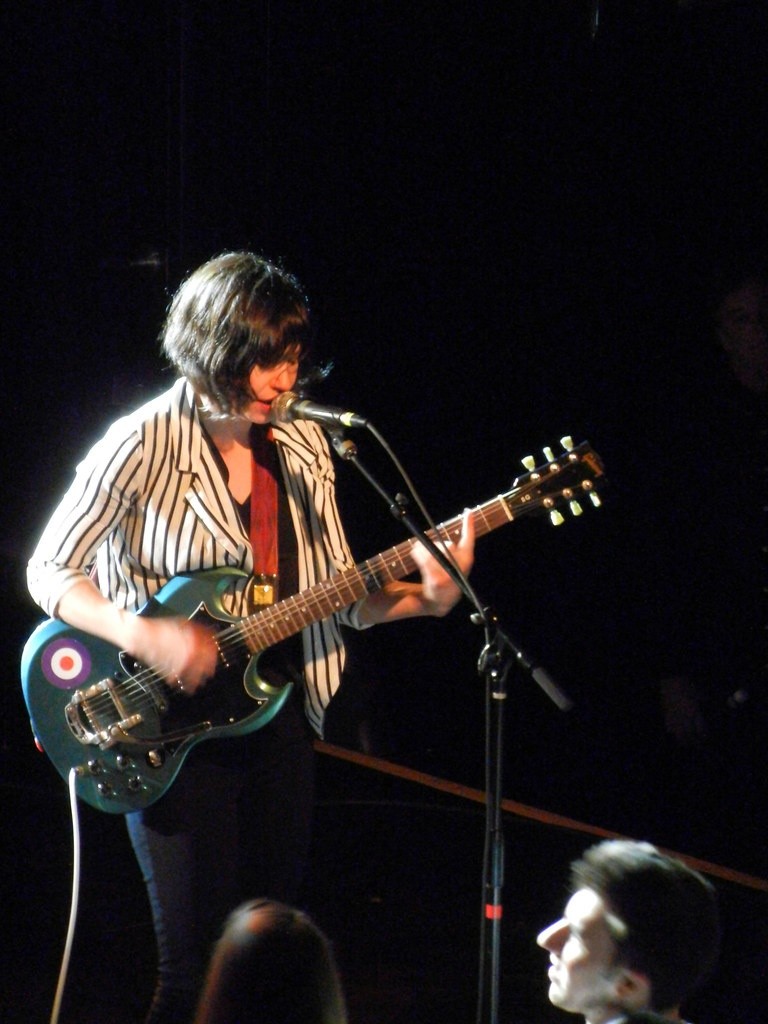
198 898 344 1024
24 251 474 1024
535 838 718 1024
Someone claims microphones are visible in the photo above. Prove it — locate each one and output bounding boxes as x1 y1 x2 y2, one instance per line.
270 391 368 426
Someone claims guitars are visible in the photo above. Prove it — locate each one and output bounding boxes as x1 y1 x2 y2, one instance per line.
20 436 607 814
